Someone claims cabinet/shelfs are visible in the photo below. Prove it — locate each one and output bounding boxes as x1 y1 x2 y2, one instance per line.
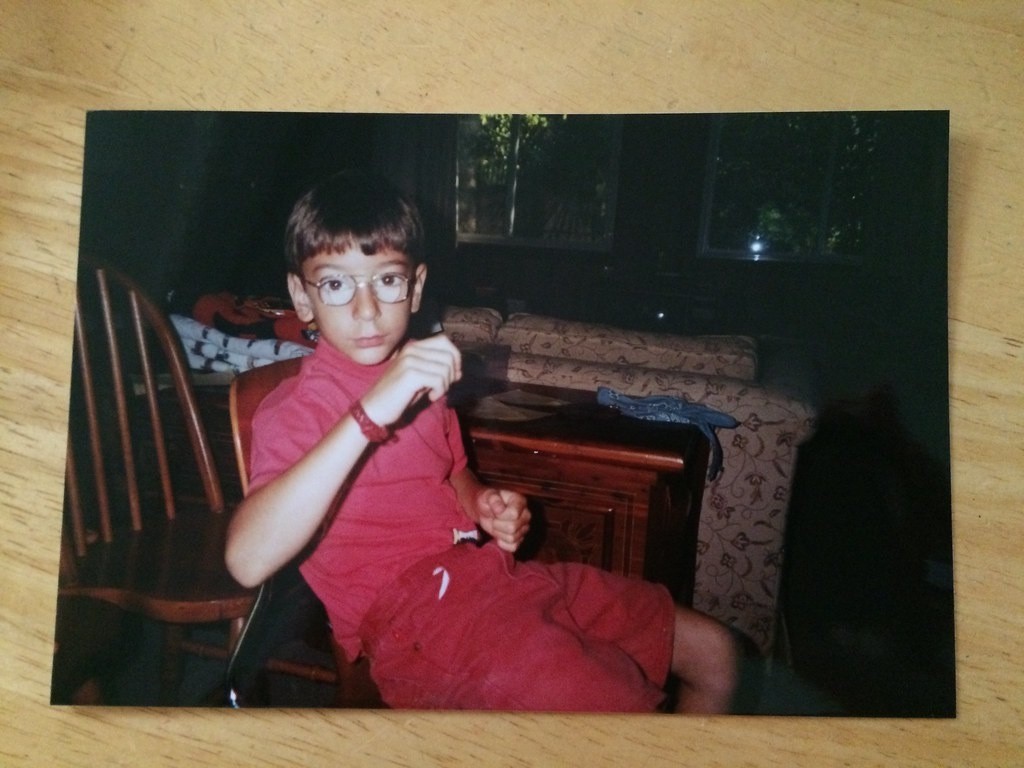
451 373 706 604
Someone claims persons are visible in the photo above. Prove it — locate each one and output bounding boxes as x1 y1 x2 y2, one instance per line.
225 177 742 715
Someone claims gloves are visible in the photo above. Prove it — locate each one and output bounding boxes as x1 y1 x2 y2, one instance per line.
597 385 736 482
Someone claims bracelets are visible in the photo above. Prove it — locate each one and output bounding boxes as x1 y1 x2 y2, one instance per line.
349 400 391 442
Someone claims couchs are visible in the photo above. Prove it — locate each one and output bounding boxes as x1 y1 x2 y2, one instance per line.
431 300 819 654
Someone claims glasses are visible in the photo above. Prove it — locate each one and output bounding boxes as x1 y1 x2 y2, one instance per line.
302 265 423 306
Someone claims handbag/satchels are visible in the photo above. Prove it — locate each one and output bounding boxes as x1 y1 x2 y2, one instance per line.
194 293 320 350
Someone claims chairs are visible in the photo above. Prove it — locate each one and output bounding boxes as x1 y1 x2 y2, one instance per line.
226 349 351 708
50 252 254 707
